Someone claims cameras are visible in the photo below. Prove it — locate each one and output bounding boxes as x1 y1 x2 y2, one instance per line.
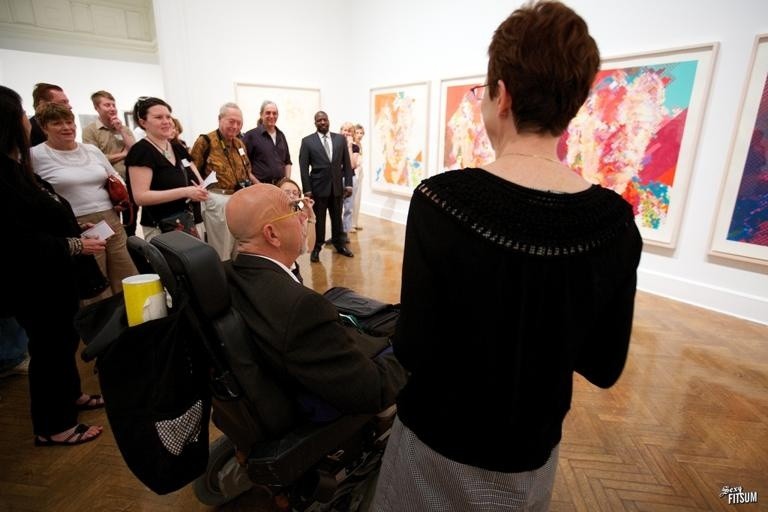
234 179 252 192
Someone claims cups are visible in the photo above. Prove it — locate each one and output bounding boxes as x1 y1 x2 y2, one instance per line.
121 272 166 328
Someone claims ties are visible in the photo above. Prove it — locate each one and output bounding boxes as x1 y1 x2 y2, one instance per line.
322 135 331 163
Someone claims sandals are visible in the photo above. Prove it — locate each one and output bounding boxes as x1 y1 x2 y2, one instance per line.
33 421 103 446
73 390 106 411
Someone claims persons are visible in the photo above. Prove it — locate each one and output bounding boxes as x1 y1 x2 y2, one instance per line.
81 90 136 183
29 83 71 146
27 102 140 307
0 85 107 447
370 4 643 512
223 183 407 432
124 96 365 260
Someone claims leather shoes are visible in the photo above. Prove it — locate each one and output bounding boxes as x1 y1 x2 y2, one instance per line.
336 247 354 258
310 244 321 262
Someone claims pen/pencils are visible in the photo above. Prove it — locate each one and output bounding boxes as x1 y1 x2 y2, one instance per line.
190 179 211 199
81 232 93 239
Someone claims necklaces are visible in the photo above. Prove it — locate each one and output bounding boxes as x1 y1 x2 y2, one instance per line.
499 152 563 167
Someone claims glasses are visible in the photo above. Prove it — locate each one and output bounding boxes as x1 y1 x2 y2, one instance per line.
469 83 491 98
270 205 304 225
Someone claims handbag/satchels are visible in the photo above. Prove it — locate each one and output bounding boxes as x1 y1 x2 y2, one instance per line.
321 286 401 354
157 209 199 242
105 174 131 213
188 168 204 224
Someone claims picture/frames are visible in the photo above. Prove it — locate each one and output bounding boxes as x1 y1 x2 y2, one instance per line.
233 80 324 145
437 40 721 257
365 80 433 201
703 32 768 275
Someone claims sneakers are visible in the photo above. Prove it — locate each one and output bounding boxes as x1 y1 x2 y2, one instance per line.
325 223 363 245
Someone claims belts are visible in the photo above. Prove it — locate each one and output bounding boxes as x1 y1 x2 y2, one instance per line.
207 188 235 195
262 180 280 185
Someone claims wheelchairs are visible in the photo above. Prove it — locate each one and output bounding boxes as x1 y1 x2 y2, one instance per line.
115 223 399 511
